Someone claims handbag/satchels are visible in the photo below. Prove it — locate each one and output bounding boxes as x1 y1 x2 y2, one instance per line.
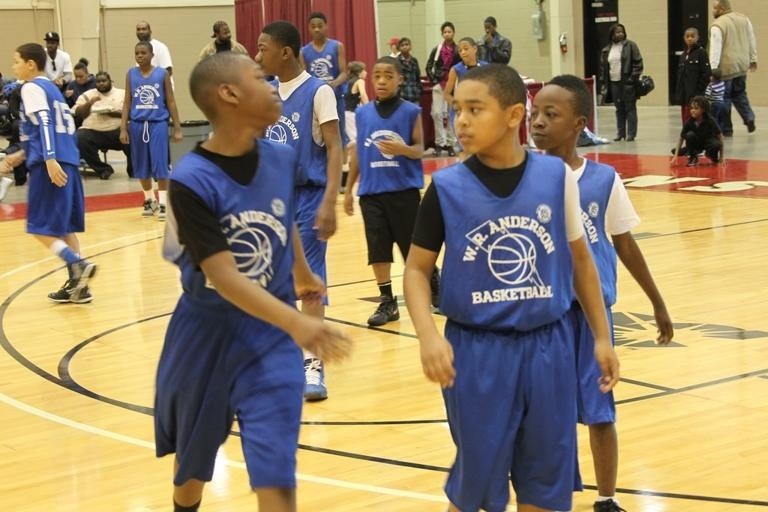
637 77 655 95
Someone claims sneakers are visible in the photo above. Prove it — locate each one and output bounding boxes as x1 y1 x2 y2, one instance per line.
448 147 455 156
432 149 440 156
303 361 327 400
687 156 698 166
144 197 157 215
369 295 400 326
158 204 166 220
747 119 755 132
431 267 442 307
47 260 97 304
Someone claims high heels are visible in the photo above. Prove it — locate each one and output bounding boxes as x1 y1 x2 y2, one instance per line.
614 136 626 141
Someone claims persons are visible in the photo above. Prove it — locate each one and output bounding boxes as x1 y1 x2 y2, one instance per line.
199 20 249 63
252 16 349 407
341 53 427 330
705 1 758 137
669 95 727 167
298 12 351 193
10 39 97 307
387 37 423 106
705 68 726 136
670 26 712 156
119 41 184 218
425 16 513 156
345 62 369 145
71 72 135 180
599 23 644 142
399 58 623 510
1 31 96 200
523 71 678 512
135 20 175 174
149 46 354 512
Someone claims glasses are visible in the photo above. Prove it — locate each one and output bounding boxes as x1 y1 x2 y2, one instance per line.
52 60 57 71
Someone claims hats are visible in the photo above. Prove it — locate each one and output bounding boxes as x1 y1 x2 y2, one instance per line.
45 33 59 42
